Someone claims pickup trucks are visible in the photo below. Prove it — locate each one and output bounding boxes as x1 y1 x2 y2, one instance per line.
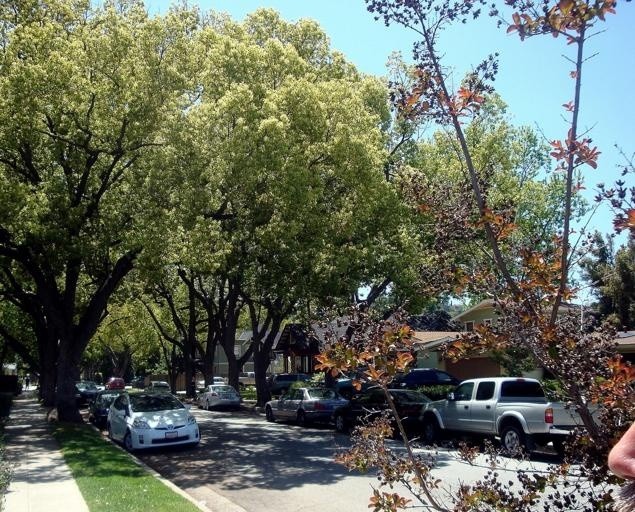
238 372 269 386
419 377 602 459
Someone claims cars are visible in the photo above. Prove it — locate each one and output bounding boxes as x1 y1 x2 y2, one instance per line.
72 376 240 453
265 368 462 439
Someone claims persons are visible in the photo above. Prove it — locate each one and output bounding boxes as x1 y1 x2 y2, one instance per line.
26 376 29 389
607 423 635 481
